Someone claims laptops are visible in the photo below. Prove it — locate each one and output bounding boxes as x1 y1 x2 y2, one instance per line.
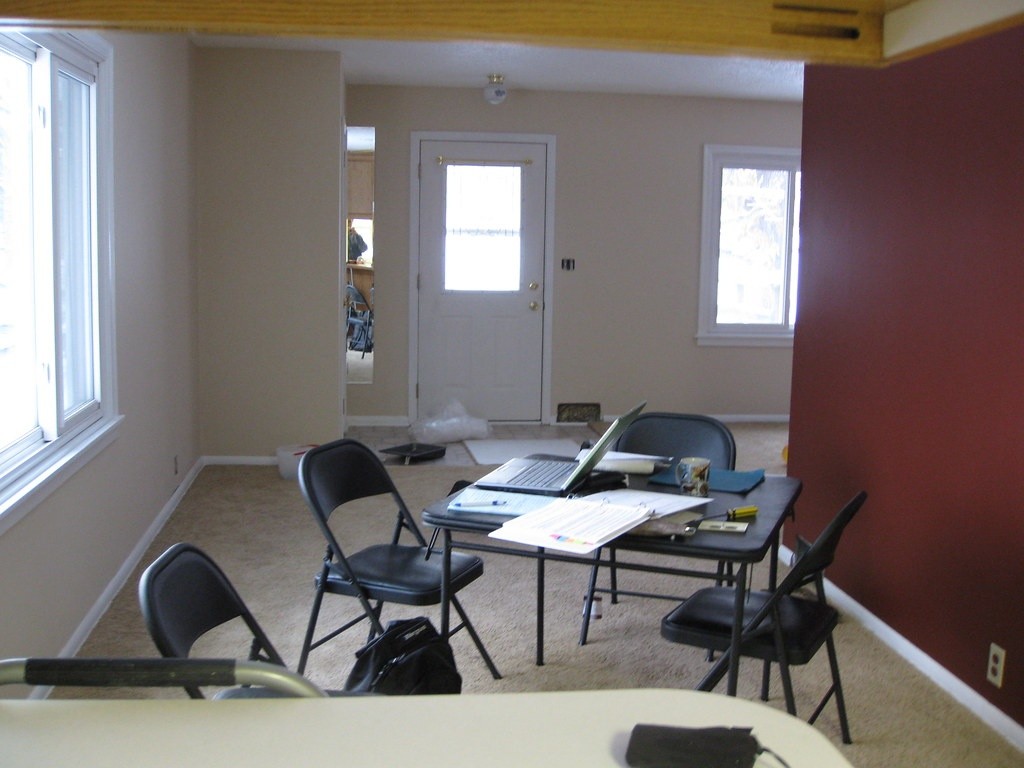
473 401 648 498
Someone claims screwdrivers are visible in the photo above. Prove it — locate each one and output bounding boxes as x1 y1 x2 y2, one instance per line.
695 505 759 523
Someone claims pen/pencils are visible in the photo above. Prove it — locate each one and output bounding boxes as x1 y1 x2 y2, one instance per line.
454 500 507 507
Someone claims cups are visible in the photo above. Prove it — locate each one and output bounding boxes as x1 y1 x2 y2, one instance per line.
675 457 711 498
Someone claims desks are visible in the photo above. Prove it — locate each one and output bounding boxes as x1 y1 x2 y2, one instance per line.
421 454 803 701
0 686 854 768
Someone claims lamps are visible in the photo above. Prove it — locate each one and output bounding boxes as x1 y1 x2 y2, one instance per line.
482 74 507 106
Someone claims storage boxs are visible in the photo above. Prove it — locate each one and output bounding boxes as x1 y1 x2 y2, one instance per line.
276 444 320 481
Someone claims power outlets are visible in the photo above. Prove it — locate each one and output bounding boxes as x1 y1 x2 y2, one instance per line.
986 642 1006 690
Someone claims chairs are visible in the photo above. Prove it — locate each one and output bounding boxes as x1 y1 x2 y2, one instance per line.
577 412 737 662
139 542 386 700
345 283 374 357
661 492 869 745
298 438 503 680
